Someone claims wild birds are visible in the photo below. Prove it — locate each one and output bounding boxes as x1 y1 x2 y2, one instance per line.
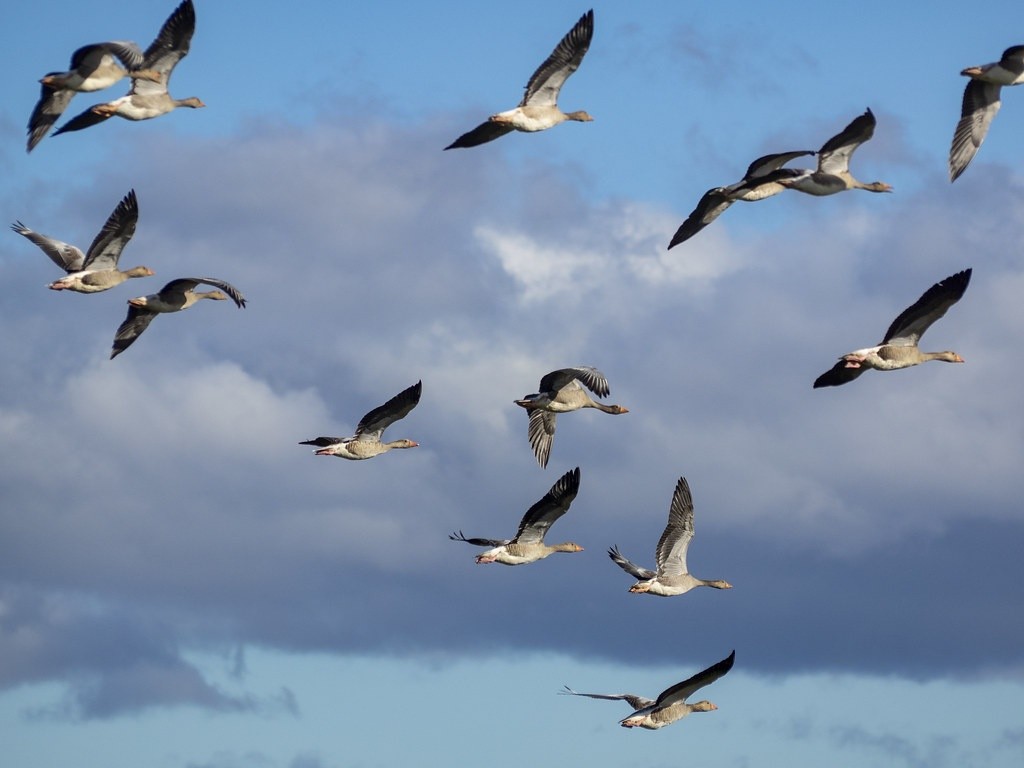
606 476 733 597
667 150 818 251
448 466 585 566
442 8 595 151
812 267 972 389
9 187 156 294
947 43 1024 185
25 40 163 155
513 365 629 470
49 0 207 138
556 648 737 730
728 107 895 196
109 278 249 362
298 379 422 460
